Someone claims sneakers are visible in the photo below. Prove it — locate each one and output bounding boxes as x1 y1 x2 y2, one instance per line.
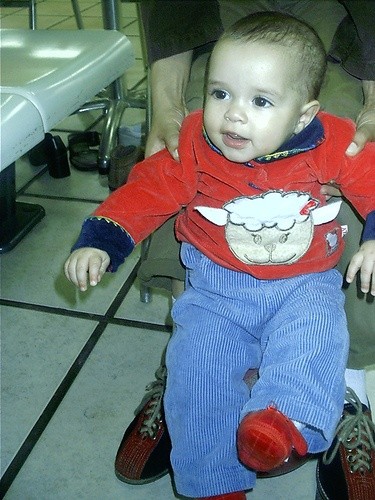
317 402 375 500
114 373 172 484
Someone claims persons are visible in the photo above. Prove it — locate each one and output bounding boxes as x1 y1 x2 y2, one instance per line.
63 10 375 500
116 1 375 500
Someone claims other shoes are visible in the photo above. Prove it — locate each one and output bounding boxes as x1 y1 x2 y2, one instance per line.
200 491 248 500
66 132 100 171
235 407 308 472
108 145 140 191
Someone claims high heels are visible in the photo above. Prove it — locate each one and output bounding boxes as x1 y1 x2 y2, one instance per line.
29 132 71 178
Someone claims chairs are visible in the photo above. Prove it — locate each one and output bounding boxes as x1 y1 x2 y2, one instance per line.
0 28 135 252
